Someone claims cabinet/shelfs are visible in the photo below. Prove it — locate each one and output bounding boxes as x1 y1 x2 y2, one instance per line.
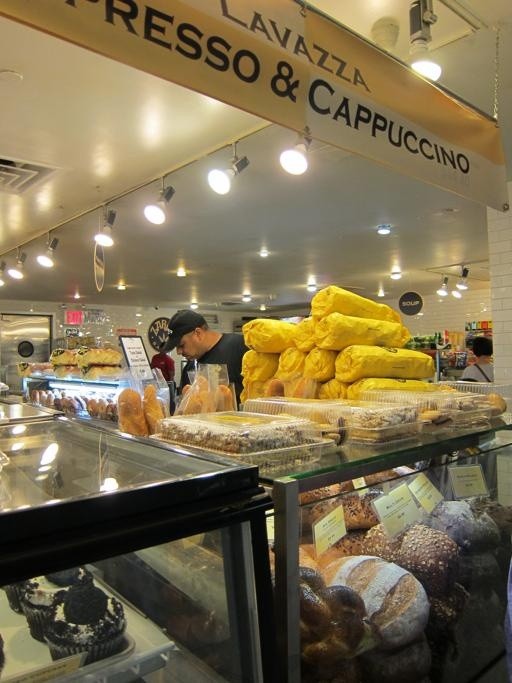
0 396 272 683
154 415 511 683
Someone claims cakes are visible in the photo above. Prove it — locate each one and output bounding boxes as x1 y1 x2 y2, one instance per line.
450 557 504 597
302 670 367 683
42 582 129 668
8 584 32 615
471 498 512 539
16 561 97 648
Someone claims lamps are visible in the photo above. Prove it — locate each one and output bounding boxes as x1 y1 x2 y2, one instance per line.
0 261 6 286
436 274 448 296
280 134 312 175
8 247 27 280
408 1 442 85
376 224 393 237
207 142 250 195
455 265 470 290
94 204 118 246
36 232 60 268
143 175 177 225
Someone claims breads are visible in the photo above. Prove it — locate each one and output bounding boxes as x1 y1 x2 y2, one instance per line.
40 390 47 405
173 375 237 414
78 347 123 367
55 390 62 399
49 349 79 365
106 404 118 420
98 400 106 418
432 500 503 553
143 386 165 435
368 639 437 681
299 567 366 671
31 389 39 405
308 490 405 535
118 389 149 438
47 390 55 406
438 599 508 681
317 555 431 654
86 400 98 418
58 400 85 415
360 523 461 597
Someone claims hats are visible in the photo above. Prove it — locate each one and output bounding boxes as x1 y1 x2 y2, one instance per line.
160 310 206 353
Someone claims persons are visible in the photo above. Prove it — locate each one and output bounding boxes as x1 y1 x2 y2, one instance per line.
150 353 174 380
461 336 499 503
159 311 246 409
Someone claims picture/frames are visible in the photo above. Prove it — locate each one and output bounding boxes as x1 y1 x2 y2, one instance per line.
118 334 156 382
